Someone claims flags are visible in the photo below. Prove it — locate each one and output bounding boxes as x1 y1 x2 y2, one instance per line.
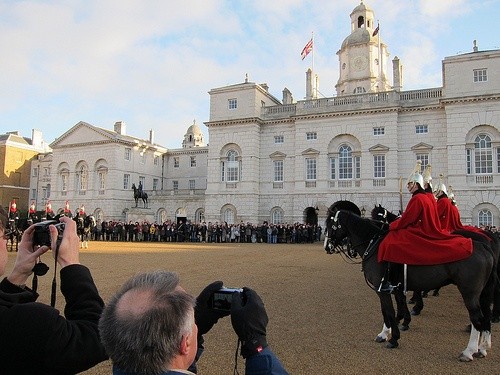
372 24 379 37
300 38 313 59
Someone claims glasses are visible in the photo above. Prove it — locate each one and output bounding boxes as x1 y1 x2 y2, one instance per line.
0 229 16 239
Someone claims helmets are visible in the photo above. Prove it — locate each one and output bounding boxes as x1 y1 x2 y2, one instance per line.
453 200 457 204
406 159 426 190
422 163 433 193
447 185 454 200
433 173 448 198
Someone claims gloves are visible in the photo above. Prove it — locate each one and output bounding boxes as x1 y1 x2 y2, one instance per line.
193 281 231 335
231 287 269 359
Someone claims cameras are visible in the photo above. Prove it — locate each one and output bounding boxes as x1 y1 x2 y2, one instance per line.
34 220 66 248
212 286 243 312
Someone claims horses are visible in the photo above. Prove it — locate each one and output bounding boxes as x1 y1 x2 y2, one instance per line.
131 183 148 208
372 202 500 321
324 206 497 363
5 213 97 252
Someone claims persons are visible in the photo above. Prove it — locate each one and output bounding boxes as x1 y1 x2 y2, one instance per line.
91 218 322 243
97 268 290 375
377 161 492 293
9 198 87 232
475 222 500 244
0 214 109 375
138 180 142 198
